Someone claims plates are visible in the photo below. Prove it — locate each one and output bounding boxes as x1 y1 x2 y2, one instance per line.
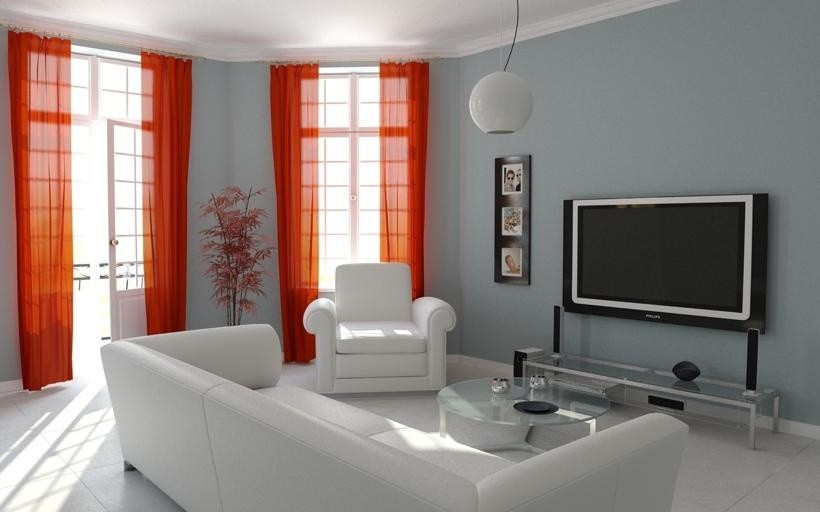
524 402 550 412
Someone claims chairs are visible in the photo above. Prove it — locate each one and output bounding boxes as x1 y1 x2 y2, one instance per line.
303 262 457 395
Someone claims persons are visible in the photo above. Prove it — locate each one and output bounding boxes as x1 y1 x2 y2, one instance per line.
513 170 522 192
503 170 516 191
504 255 521 274
504 207 521 233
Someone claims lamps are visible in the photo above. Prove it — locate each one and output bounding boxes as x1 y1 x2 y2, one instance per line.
469 0 532 135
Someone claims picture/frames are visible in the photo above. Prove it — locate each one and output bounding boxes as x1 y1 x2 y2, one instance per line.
494 154 532 286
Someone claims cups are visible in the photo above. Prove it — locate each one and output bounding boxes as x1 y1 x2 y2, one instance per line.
492 377 511 394
530 375 548 390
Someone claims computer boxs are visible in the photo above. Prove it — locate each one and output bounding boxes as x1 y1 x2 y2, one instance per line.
512 347 544 386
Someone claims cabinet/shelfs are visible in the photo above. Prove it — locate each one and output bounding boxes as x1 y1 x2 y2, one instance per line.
522 352 779 450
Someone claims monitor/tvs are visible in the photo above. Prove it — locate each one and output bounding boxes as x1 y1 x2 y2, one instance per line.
570 193 755 322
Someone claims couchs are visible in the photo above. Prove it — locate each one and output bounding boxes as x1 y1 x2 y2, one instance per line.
100 324 690 512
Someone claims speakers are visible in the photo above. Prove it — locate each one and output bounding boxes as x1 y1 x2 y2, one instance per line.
552 304 565 360
741 326 761 397
